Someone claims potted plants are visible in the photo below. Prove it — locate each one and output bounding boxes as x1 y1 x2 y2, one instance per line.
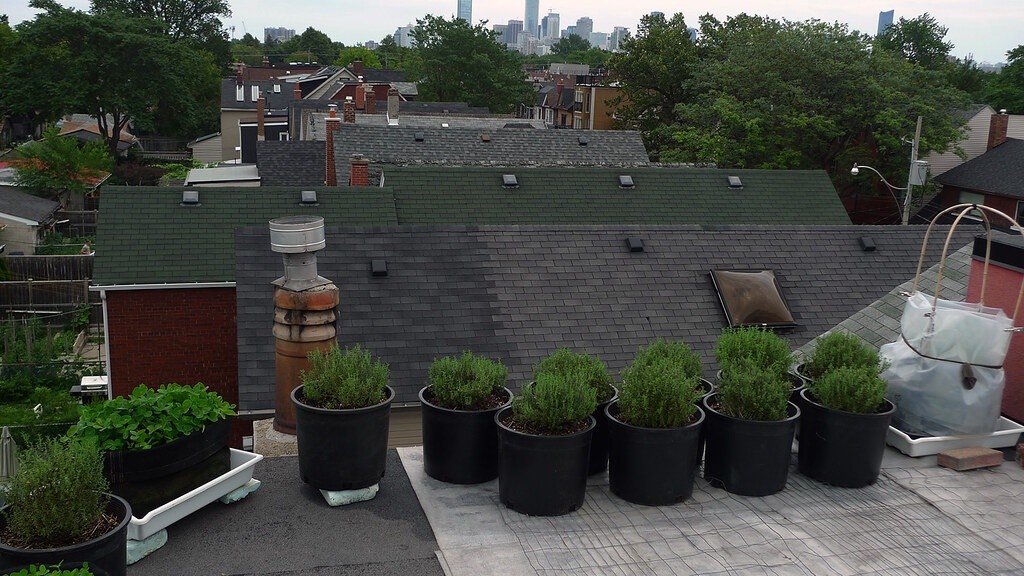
421 344 517 486
798 362 899 488
605 352 707 508
281 335 399 489
631 339 713 405
493 369 600 518
795 332 893 382
718 327 805 403
706 355 801 497
59 363 236 516
0 423 133 576
527 347 619 478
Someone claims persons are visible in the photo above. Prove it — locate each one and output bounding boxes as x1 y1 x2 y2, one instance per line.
82 239 94 255
